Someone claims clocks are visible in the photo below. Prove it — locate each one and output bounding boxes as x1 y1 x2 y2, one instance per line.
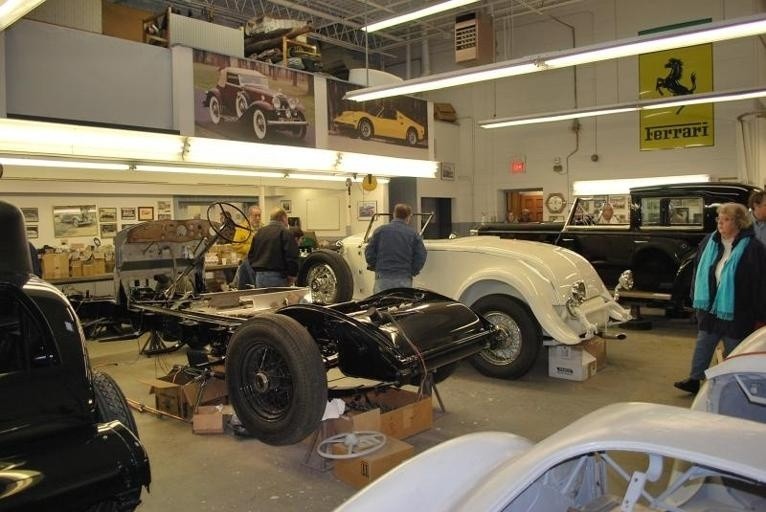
544 193 567 214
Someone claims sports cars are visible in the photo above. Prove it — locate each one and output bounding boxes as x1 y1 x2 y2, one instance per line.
333 99 427 146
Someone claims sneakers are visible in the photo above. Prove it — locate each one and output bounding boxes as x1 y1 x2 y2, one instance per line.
673 378 700 394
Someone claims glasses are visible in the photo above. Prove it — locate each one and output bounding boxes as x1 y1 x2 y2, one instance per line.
715 217 734 223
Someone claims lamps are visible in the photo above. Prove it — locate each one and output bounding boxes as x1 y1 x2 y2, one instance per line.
341 10 766 104
475 30 766 133
361 1 479 36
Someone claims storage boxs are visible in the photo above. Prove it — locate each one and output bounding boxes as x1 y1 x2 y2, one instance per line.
145 359 233 434
545 334 609 382
33 240 244 294
325 386 433 492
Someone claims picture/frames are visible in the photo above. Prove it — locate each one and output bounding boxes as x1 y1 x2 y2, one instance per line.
136 206 155 222
440 160 456 182
97 206 118 222
100 222 118 239
279 199 292 213
355 201 378 222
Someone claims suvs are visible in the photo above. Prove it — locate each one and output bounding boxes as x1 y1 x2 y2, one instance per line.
200 62 311 141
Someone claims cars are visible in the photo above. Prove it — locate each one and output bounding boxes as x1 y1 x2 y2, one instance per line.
0 199 165 511
60 191 505 446
324 210 638 380
460 179 766 315
324 400 765 512
59 212 93 228
659 319 766 511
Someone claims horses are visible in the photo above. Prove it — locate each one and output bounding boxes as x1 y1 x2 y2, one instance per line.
655 57 697 113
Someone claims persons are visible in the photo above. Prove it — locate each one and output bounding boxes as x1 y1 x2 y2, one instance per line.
232 206 267 267
598 204 624 224
364 203 427 294
231 259 256 290
248 206 300 289
750 191 765 247
218 211 233 244
675 202 766 393
